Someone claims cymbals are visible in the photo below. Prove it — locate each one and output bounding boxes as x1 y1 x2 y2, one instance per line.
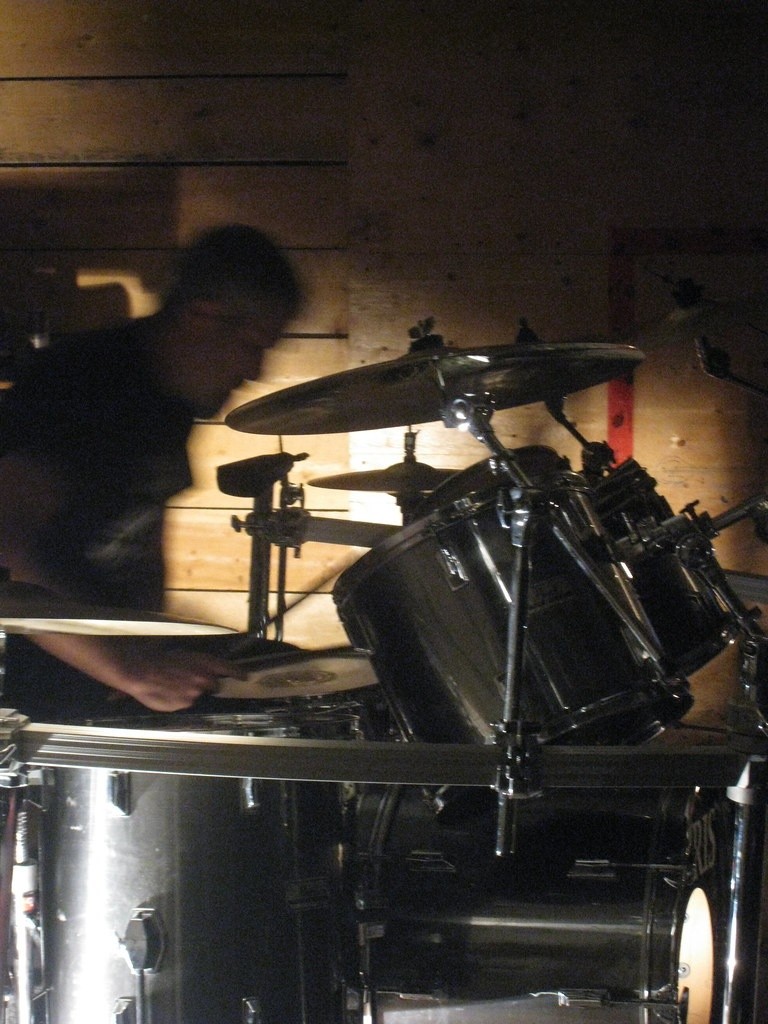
222 342 642 436
305 464 462 493
626 288 768 349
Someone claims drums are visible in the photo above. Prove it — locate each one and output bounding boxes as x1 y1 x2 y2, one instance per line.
203 649 409 742
411 445 730 675
38 713 368 1023
337 782 768 1024
332 470 694 826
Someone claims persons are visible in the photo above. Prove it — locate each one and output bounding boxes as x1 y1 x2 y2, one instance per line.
0 222 307 1024
